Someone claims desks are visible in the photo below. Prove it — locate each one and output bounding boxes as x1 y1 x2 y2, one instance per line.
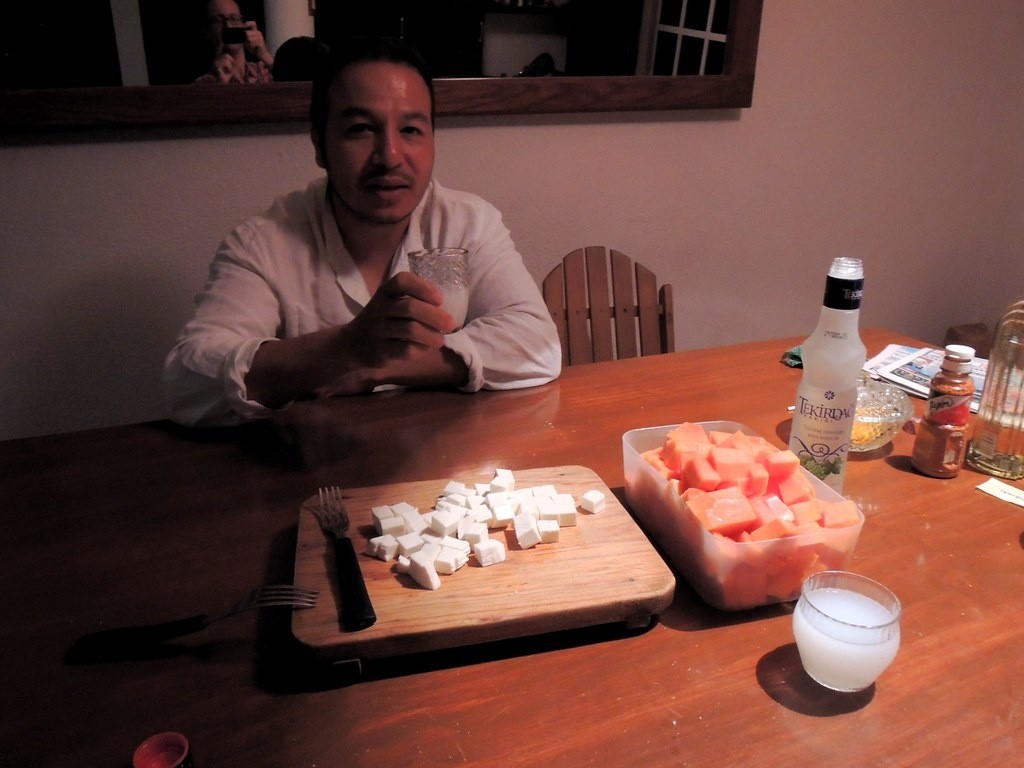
0 325 1024 768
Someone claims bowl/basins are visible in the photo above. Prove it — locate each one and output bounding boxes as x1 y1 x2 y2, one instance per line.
622 420 865 611
849 379 914 453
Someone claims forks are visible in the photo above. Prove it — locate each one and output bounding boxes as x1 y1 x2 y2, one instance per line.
317 485 378 630
75 585 319 661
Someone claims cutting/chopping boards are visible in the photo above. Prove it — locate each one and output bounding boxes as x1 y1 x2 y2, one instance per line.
291 465 676 661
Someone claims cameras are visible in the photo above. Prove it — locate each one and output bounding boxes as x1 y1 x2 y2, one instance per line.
221 26 251 45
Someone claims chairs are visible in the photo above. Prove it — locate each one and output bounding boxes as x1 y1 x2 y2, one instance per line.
541 245 675 367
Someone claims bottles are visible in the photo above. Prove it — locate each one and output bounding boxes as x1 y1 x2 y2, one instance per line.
911 345 976 478
966 297 1024 480
789 256 866 496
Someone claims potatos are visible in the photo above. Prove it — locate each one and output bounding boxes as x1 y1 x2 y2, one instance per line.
631 422 863 610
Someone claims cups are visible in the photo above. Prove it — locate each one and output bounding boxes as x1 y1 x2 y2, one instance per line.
407 247 470 335
133 732 194 768
790 570 903 692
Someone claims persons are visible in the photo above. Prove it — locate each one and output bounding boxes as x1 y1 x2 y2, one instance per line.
159 43 562 426
169 0 274 85
